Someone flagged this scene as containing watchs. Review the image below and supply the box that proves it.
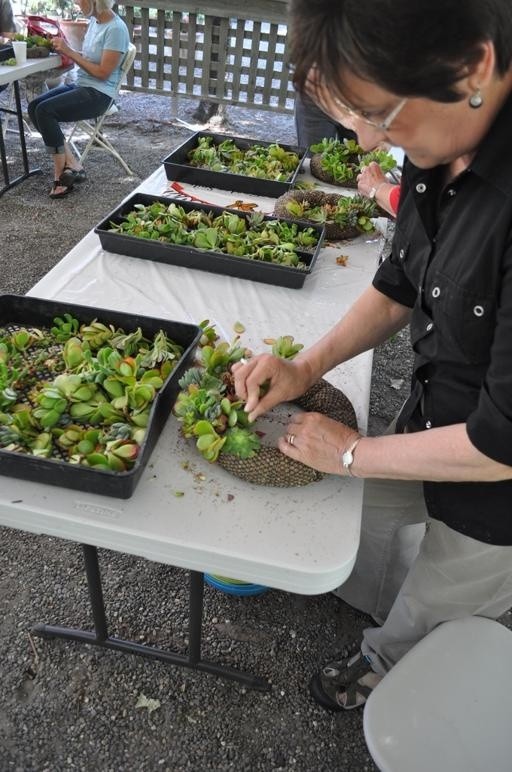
[340,439,361,478]
[369,182,382,201]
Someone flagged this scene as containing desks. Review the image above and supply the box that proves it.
[0,50,86,197]
[0,128,390,691]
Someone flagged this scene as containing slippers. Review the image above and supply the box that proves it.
[49,164,86,198]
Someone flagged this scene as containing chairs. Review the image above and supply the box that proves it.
[38,40,138,177]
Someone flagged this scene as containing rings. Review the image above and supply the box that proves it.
[287,434,295,445]
[240,358,248,365]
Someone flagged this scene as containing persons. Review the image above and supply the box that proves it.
[356,161,401,218]
[293,61,356,157]
[230,0,512,711]
[0,0,16,93]
[28,0,131,198]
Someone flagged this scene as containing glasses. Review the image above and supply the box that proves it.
[340,95,406,131]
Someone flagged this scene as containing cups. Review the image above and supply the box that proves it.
[12,41,28,65]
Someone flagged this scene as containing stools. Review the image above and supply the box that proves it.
[363,611,512,767]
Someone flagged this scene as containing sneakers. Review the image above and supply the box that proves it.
[310,651,381,711]
